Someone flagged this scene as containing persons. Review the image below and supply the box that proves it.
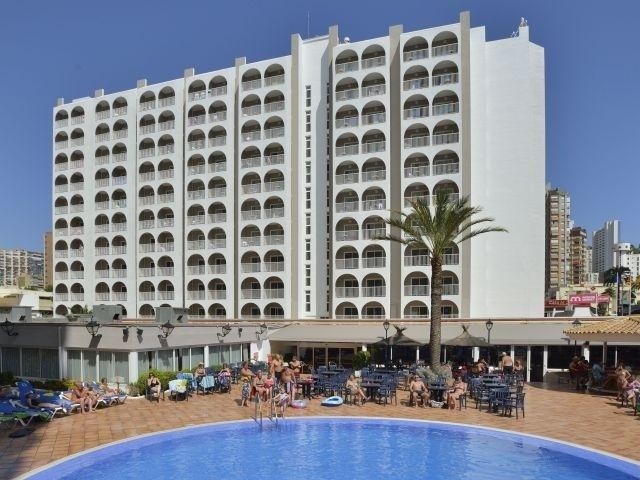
[448,376,467,410]
[409,374,428,409]
[25,393,60,408]
[147,372,161,404]
[346,374,370,405]
[456,352,526,384]
[562,355,640,411]
[275,388,290,419]
[71,376,126,414]
[195,354,302,406]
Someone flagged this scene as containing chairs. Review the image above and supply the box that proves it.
[236,360,527,419]
[622,386,640,416]
[0,364,236,433]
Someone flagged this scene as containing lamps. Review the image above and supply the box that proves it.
[85,315,100,338]
[0,317,19,336]
[256,322,267,335]
[217,322,232,337]
[158,320,175,339]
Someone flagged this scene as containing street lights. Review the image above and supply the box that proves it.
[383,317,390,362]
[486,317,493,364]
[572,318,582,389]
